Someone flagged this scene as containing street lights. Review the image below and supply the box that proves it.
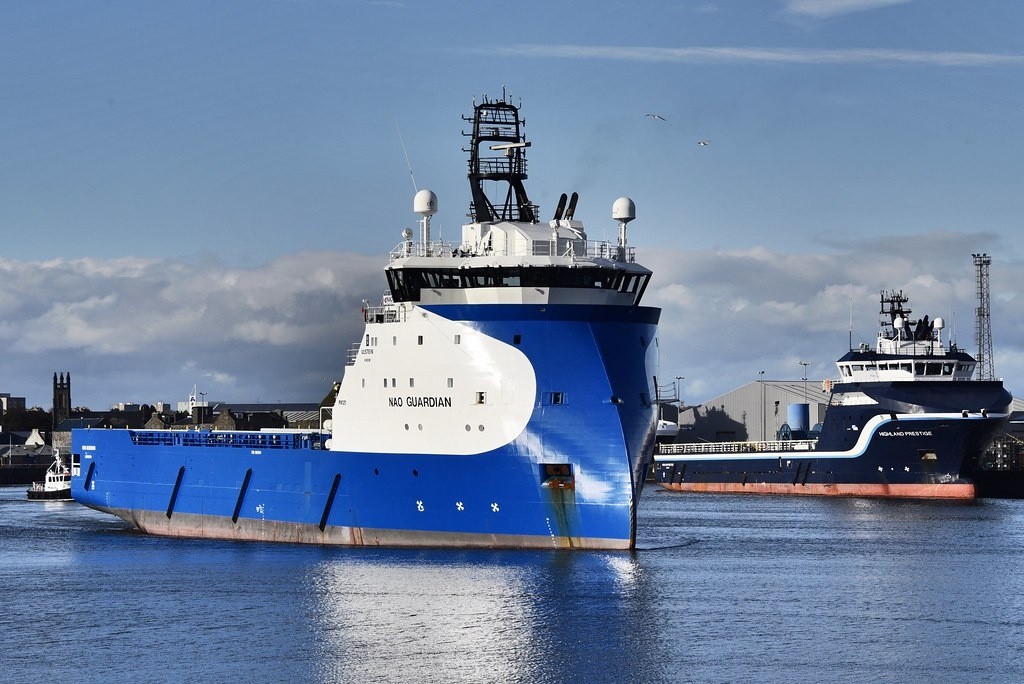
[757,370,766,442]
[199,392,208,430]
[798,360,812,402]
[673,375,684,443]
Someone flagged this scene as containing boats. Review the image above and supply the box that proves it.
[25,446,76,503]
[652,290,1013,504]
[68,81,669,557]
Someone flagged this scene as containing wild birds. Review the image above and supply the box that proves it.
[698,141,707,146]
[646,114,667,121]
